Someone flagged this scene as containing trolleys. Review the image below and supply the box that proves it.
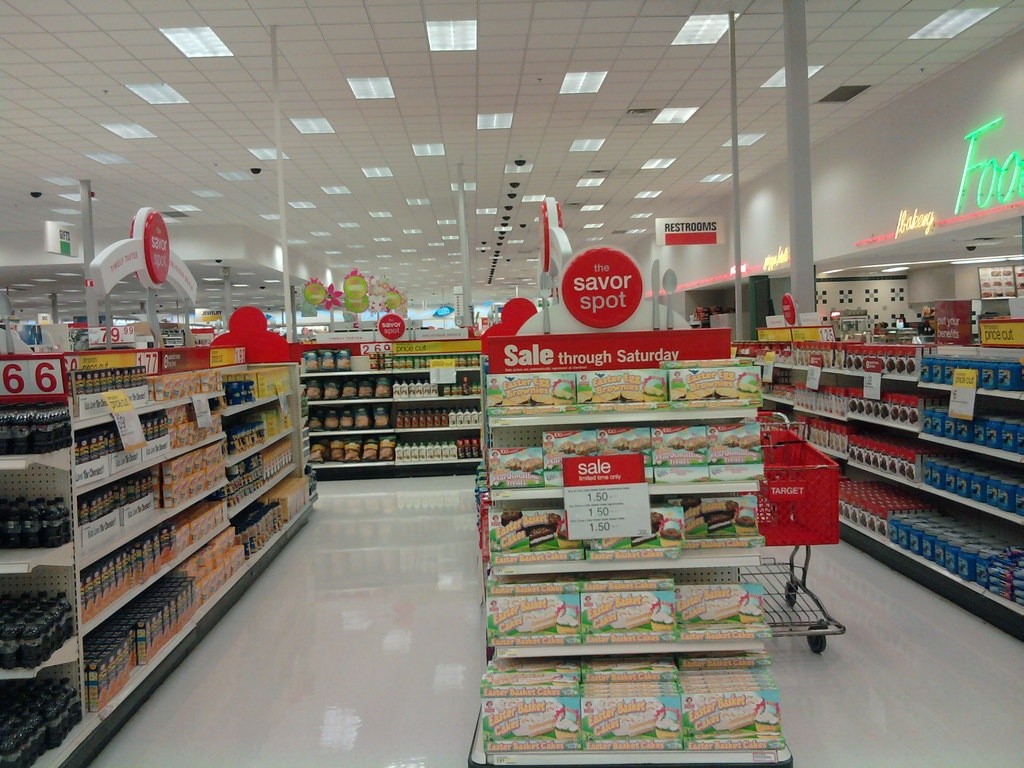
[737,412,846,654]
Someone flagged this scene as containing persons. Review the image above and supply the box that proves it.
[545,435,554,446]
[673,372,683,382]
[708,428,717,439]
[584,701,594,713]
[653,430,662,442]
[486,701,495,712]
[597,431,607,443]
[491,515,500,526]
[490,452,500,462]
[584,596,593,607]
[685,697,695,708]
[675,589,682,598]
[489,601,499,611]
[490,379,499,390]
[579,376,589,385]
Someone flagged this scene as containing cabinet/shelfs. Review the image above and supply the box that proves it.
[221,390,315,584]
[753,360,1024,641]
[300,370,394,481]
[247,363,318,505]
[393,368,483,478]
[0,447,86,768]
[72,389,251,733]
[466,405,793,768]
